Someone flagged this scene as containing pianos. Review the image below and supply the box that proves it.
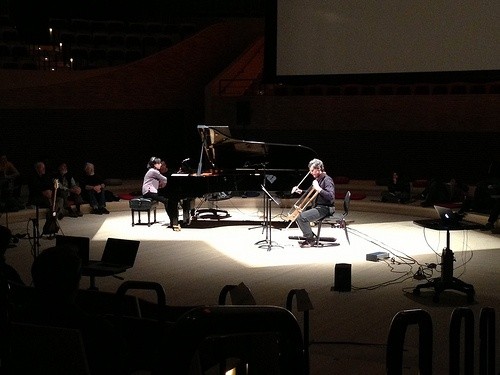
[166,125,316,233]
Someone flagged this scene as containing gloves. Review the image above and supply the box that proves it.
[312,180,322,193]
[291,186,302,194]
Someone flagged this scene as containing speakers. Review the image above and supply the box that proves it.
[335,263,352,292]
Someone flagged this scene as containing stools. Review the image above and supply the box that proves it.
[129,196,157,227]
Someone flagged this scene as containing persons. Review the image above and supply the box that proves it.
[16,245,128,375]
[28,162,64,219]
[80,163,109,214]
[142,157,184,228]
[291,159,336,248]
[49,162,83,217]
[383,171,402,203]
[0,226,26,318]
[0,152,25,211]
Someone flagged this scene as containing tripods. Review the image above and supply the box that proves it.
[247,174,284,249]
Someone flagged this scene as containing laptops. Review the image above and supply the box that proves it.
[434,205,471,225]
[90,238,141,269]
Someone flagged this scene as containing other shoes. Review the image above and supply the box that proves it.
[92,208,102,215]
[77,210,83,216]
[101,207,109,214]
[68,210,77,218]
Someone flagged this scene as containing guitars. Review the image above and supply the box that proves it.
[42,180,61,238]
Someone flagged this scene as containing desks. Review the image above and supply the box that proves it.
[415,219,486,301]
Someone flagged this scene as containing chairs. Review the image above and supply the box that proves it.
[55,236,140,292]
[317,191,351,247]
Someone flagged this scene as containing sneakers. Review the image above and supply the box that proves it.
[300,236,318,247]
